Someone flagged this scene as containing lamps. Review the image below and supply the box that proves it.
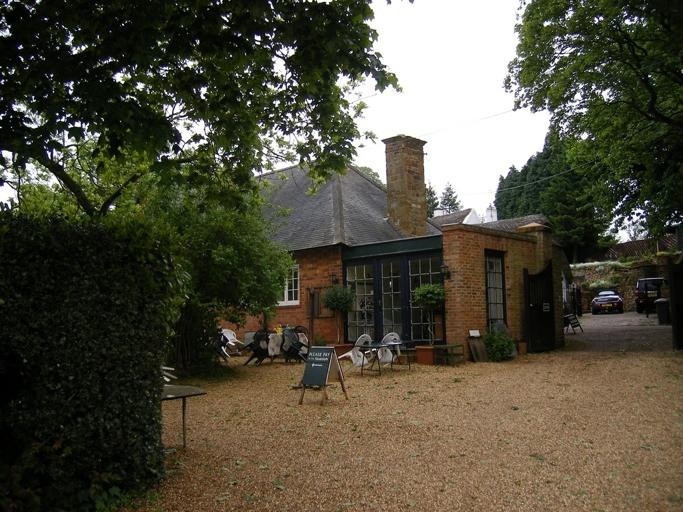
[439,264,451,279]
[329,272,339,283]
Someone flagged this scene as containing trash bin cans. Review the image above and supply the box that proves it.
[655,298,670,323]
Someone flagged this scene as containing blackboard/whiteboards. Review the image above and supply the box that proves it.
[302,346,334,386]
[567,314,579,327]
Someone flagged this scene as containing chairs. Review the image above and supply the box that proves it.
[215,325,311,367]
[337,332,403,376]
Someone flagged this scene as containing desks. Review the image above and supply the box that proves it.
[350,339,414,374]
